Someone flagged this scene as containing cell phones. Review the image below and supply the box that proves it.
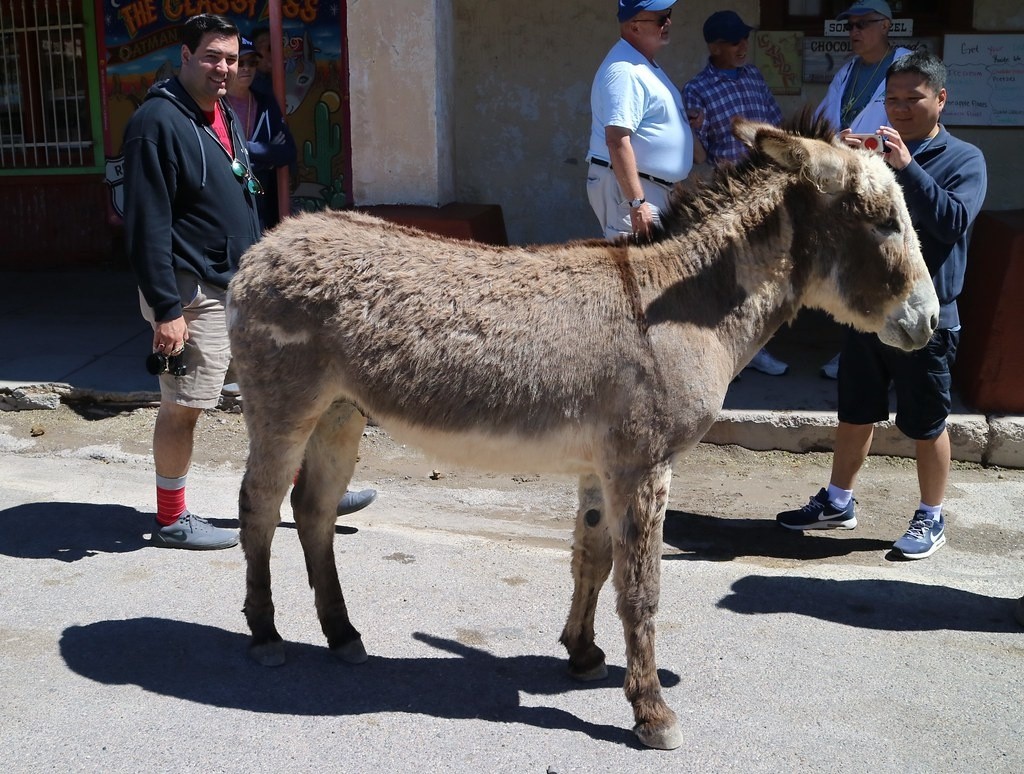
[847,133,884,153]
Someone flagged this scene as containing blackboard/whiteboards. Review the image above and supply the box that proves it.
[937,28,1024,130]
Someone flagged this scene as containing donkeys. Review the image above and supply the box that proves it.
[223,103,941,752]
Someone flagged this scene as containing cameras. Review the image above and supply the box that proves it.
[146,350,186,375]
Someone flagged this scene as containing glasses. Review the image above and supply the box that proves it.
[631,8,672,27]
[714,32,750,46]
[238,59,259,67]
[843,19,886,31]
[231,158,264,194]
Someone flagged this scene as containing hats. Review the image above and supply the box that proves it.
[239,37,262,57]
[703,11,754,41]
[617,0,678,23]
[837,0,892,21]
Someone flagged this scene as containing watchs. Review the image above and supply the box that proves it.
[629,197,645,208]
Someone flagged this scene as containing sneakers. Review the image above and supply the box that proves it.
[291,489,376,523]
[152,509,240,550]
[776,488,858,530]
[891,510,945,558]
[820,352,840,379]
[744,346,790,375]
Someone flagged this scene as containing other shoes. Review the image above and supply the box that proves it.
[221,375,241,395]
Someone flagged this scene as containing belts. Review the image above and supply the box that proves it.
[591,156,673,187]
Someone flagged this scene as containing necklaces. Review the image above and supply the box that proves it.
[844,41,889,121]
[226,90,251,141]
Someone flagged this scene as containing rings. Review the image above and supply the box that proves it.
[159,344,166,349]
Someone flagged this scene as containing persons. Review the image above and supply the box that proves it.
[681,10,788,374]
[586,0,704,240]
[222,32,294,395]
[775,52,987,559]
[122,13,376,550]
[811,0,915,380]
